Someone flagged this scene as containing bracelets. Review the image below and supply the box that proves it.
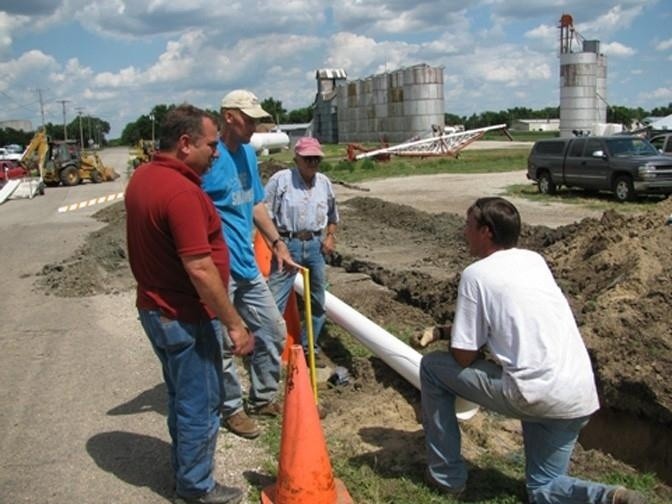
[272,238,283,246]
[435,325,444,340]
[326,232,337,239]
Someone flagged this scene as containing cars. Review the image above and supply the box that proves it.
[0,143,26,180]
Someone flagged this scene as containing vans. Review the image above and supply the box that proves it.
[527,136,672,201]
[643,134,672,157]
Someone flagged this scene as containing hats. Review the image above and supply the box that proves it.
[294,137,324,156]
[221,89,272,119]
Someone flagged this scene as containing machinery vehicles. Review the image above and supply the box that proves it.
[20,131,120,185]
[130,138,160,169]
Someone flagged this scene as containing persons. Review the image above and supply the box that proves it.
[410,194,644,504]
[197,88,289,437]
[263,136,339,369]
[124,104,243,504]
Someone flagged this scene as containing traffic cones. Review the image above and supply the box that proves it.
[254,227,301,363]
[261,348,355,504]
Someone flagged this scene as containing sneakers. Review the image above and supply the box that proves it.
[172,477,241,504]
[424,468,466,492]
[247,403,280,416]
[224,411,259,438]
[612,488,645,504]
[305,355,325,368]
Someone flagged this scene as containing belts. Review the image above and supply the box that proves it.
[280,232,320,240]
